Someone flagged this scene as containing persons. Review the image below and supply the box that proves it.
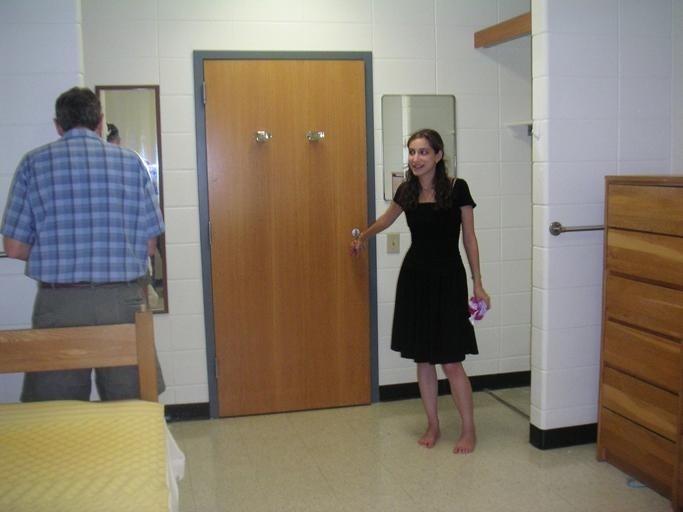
[352,130,490,455]
[106,123,158,202]
[0,87,166,402]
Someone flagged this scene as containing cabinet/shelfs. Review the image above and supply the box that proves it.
[593,174,682,506]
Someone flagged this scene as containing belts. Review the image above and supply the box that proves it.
[41,281,90,288]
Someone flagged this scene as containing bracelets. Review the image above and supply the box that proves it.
[471,273,480,280]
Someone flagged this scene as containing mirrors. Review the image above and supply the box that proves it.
[381,94,458,201]
[96,84,170,316]
